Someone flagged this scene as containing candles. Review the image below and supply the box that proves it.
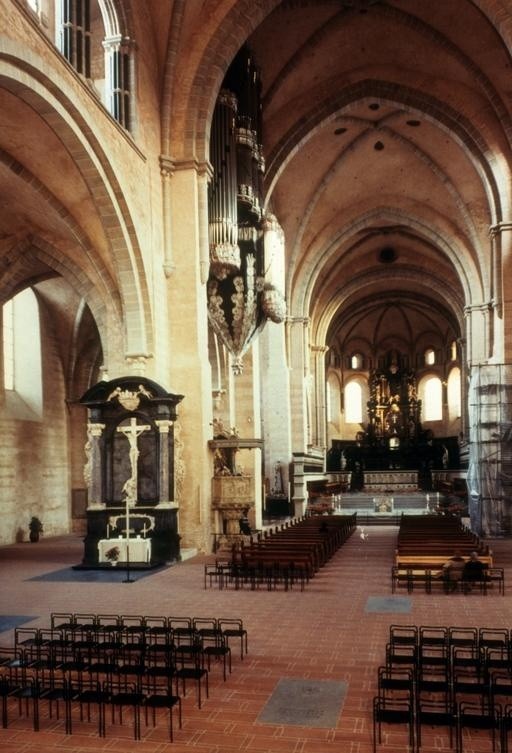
[106,524,110,540]
[125,494,130,549]
[143,522,147,537]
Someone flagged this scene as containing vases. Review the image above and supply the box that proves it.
[110,560,118,566]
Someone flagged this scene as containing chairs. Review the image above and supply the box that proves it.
[201,505,361,595]
[368,623,512,752]
[390,507,507,596]
[0,611,249,743]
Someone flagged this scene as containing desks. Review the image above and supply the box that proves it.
[96,535,154,566]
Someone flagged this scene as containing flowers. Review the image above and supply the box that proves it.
[104,546,120,561]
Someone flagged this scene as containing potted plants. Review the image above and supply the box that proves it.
[26,512,45,543]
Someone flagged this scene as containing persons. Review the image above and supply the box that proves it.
[440,548,466,592]
[460,551,486,592]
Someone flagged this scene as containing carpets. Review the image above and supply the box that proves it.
[21,563,176,583]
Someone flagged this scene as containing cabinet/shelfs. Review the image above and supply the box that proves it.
[69,374,184,573]
